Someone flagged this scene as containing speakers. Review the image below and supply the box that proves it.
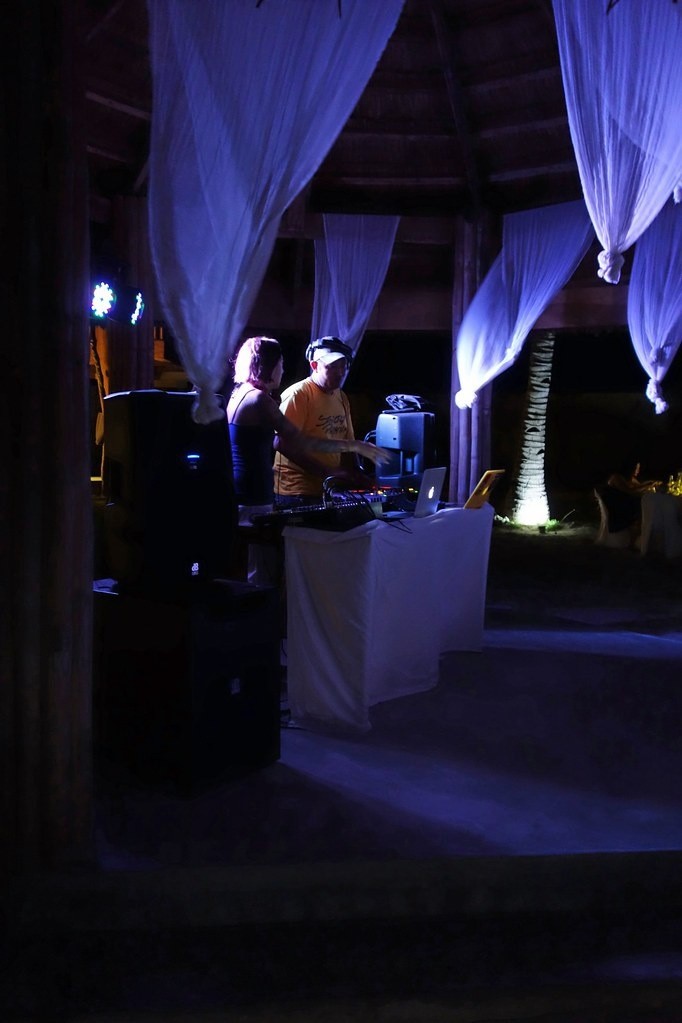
[375,412,437,492]
[103,390,236,603]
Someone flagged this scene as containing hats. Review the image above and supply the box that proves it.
[306,336,353,363]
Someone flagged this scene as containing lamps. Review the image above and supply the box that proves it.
[90,273,151,328]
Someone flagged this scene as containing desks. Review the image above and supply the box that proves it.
[282,504,491,730]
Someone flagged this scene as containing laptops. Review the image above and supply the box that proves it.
[462,469,506,509]
[383,467,446,520]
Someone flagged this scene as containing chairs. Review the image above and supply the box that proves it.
[593,483,637,546]
[639,494,682,559]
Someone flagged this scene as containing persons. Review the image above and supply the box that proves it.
[275,337,358,511]
[229,335,391,585]
[604,458,663,550]
